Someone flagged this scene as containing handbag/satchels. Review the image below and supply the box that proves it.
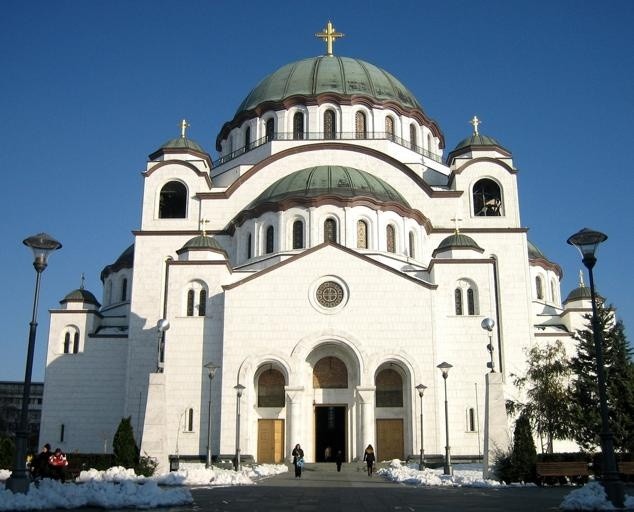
[296,457,304,467]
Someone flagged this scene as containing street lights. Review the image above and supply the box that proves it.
[415,384,427,471]
[5,232,62,495]
[437,362,453,476]
[234,384,246,472]
[204,362,221,470]
[567,227,625,508]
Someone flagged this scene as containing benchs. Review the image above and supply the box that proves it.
[607,462,634,483]
[27,457,81,483]
[536,461,590,489]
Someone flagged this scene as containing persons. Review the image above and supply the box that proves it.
[31,443,68,483]
[364,444,375,477]
[336,449,342,472]
[292,444,304,479]
[325,446,331,462]
